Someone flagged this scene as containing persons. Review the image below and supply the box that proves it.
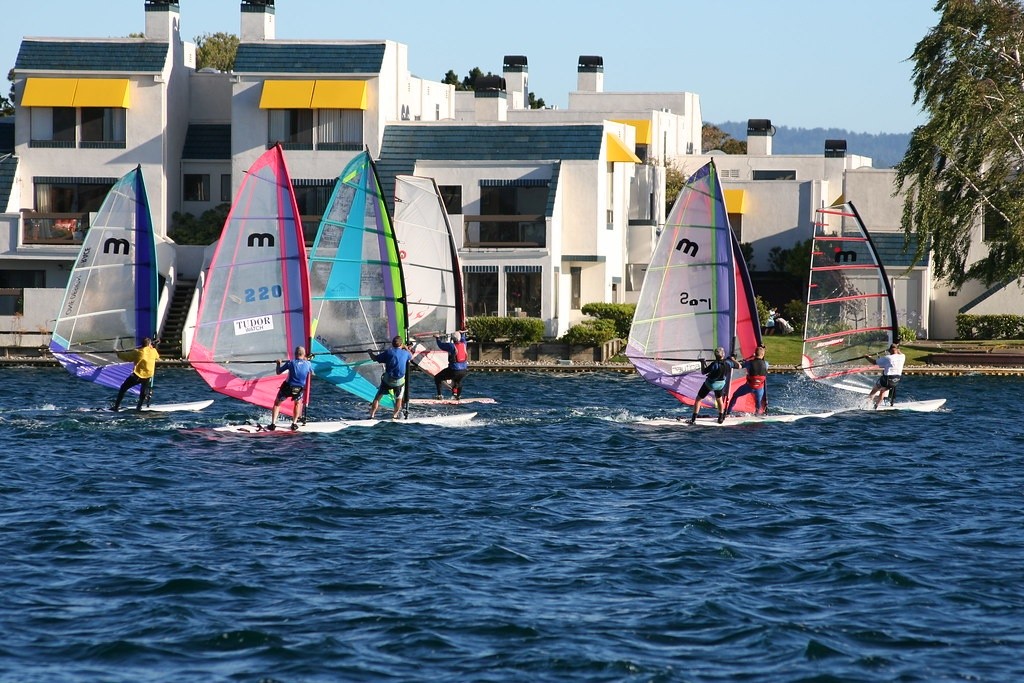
[367,336,412,420]
[432,330,469,400]
[267,346,311,430]
[764,307,780,336]
[727,346,770,415]
[685,346,742,424]
[109,337,160,412]
[863,344,906,409]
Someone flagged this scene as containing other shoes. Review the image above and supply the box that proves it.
[718,418,723,424]
[455,396,460,400]
[109,406,118,411]
[392,415,400,419]
[136,407,141,410]
[267,424,275,430]
[291,423,298,430]
[367,417,374,420]
[437,395,442,400]
[685,420,693,424]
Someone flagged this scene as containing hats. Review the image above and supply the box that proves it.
[451,331,461,341]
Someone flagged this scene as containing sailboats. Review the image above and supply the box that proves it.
[776,201,948,417]
[296,141,480,430]
[621,157,837,428]
[46,163,215,417]
[382,173,497,405]
[178,142,314,442]
